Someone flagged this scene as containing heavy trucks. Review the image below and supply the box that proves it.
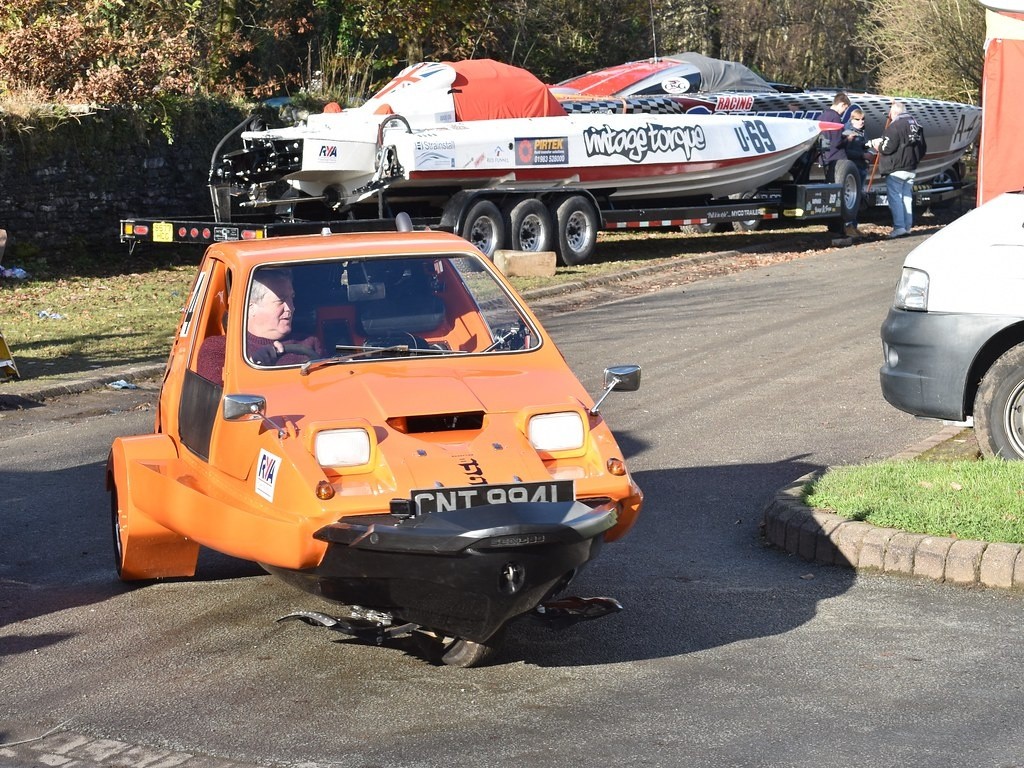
[117,49,981,269]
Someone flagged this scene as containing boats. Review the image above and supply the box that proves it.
[302,52,985,209]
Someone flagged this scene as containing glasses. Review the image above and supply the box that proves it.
[854,117,866,121]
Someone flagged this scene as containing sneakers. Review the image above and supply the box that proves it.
[844,225,860,237]
[856,230,868,238]
[906,230,911,235]
[886,228,906,238]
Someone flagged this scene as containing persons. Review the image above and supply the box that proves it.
[815,94,928,247]
[196,271,343,385]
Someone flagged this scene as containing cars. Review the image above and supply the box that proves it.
[876,185,1024,468]
